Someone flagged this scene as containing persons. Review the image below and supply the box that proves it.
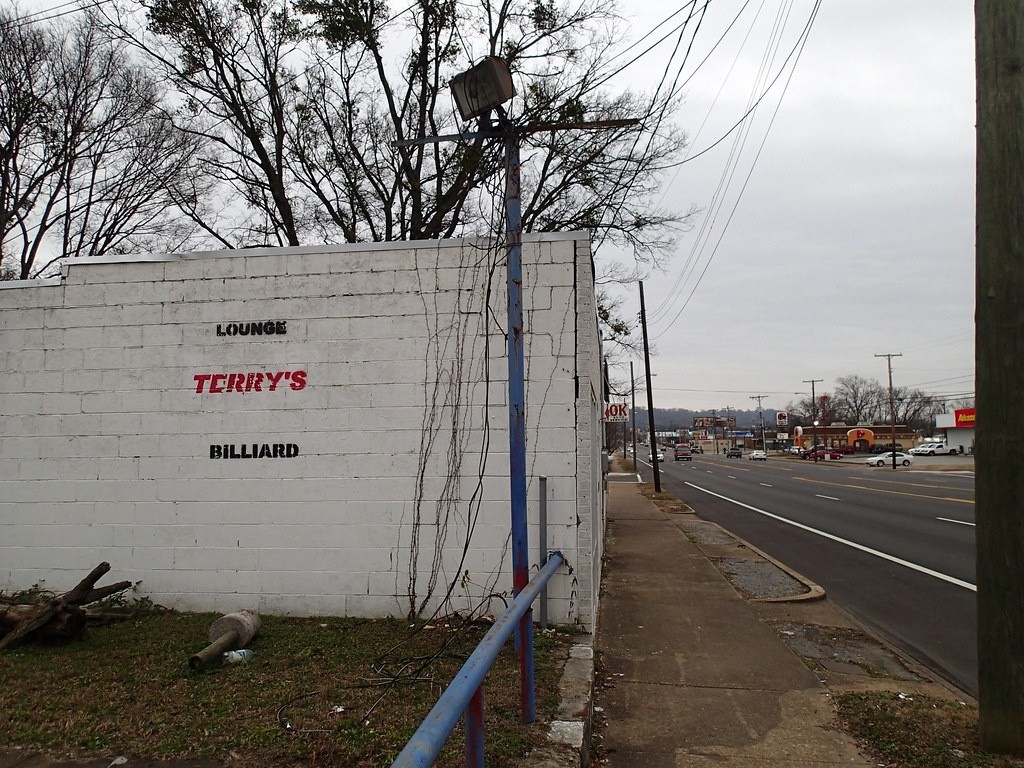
[723,448,726,454]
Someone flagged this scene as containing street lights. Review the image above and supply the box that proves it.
[630,361,658,472]
[795,392,819,462]
[448,53,532,651]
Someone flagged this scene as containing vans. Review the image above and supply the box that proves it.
[674,444,693,461]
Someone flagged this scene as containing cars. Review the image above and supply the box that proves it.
[865,451,916,467]
[648,448,665,462]
[784,440,961,461]
[748,449,767,461]
[626,442,666,454]
[726,446,742,458]
